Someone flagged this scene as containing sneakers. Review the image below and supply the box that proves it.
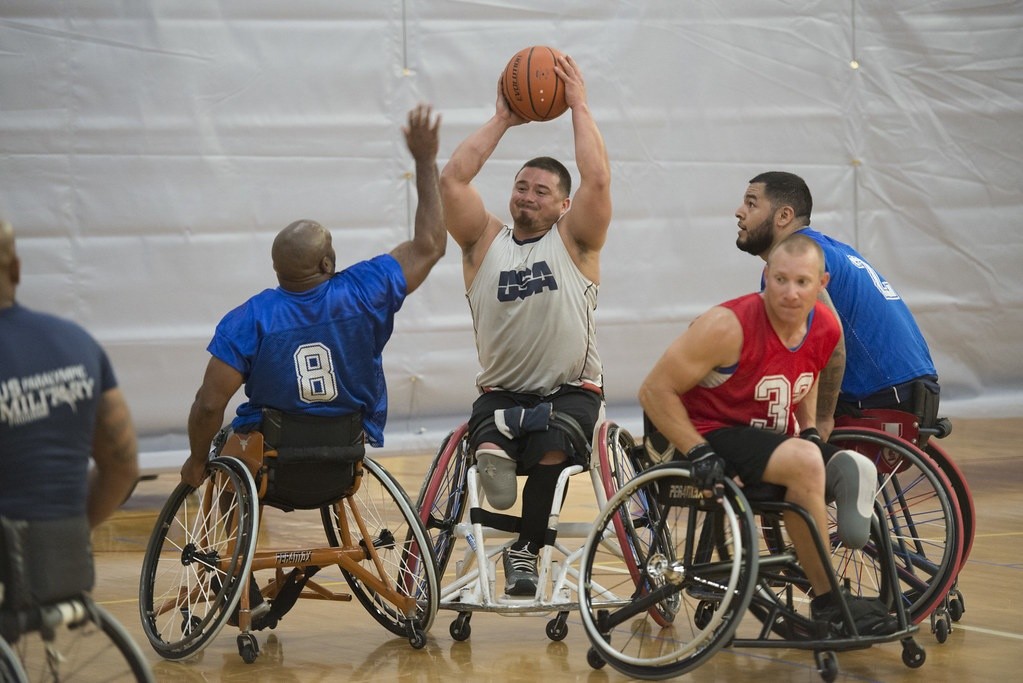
[811,586,900,638]
[503,546,540,596]
[227,571,270,626]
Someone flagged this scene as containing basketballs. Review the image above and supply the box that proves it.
[499,44,572,124]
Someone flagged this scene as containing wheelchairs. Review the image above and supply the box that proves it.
[397,376,684,641]
[0,474,158,683]
[576,378,977,683]
[139,406,441,663]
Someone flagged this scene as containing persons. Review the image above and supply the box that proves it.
[736,172,939,446]
[0,217,138,601]
[437,55,611,595]
[180,104,448,622]
[641,234,898,637]
